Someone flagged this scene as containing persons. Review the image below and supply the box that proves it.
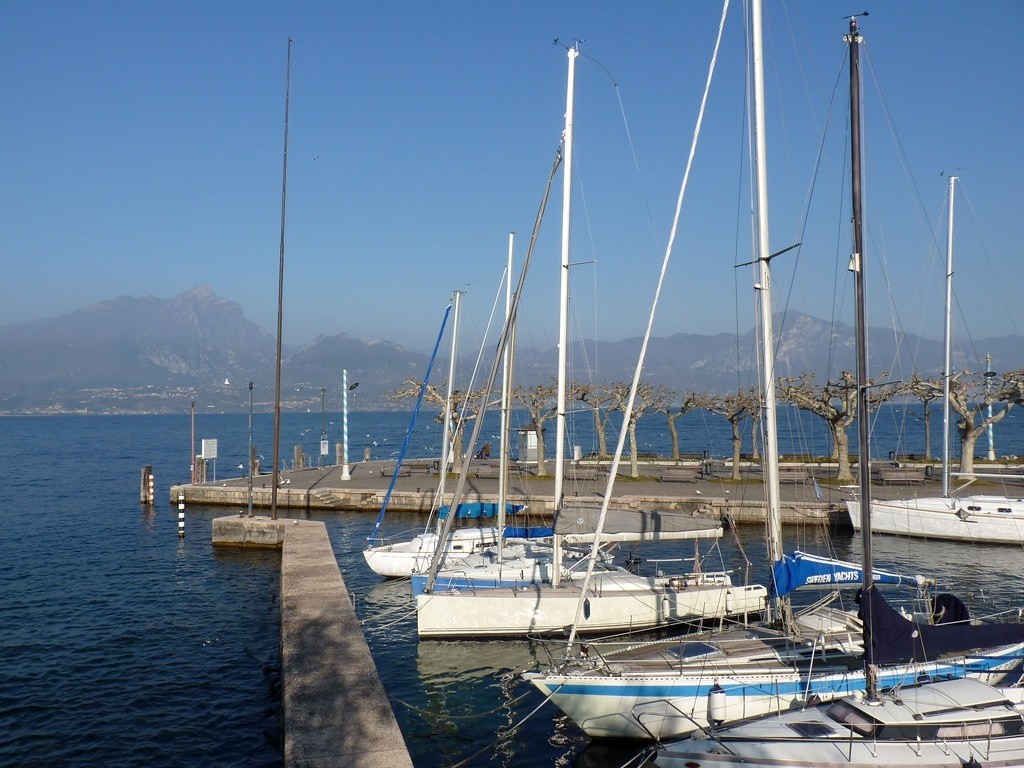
[477,440,489,459]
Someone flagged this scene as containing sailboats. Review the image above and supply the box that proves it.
[364,0,1024,767]
[844,174,1024,548]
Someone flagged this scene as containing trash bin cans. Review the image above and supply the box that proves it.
[704,451,708,460]
[888,450,894,460]
[574,446,582,461]
[926,465,933,477]
[706,463,712,475]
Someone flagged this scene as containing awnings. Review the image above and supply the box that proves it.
[438,502,528,520]
